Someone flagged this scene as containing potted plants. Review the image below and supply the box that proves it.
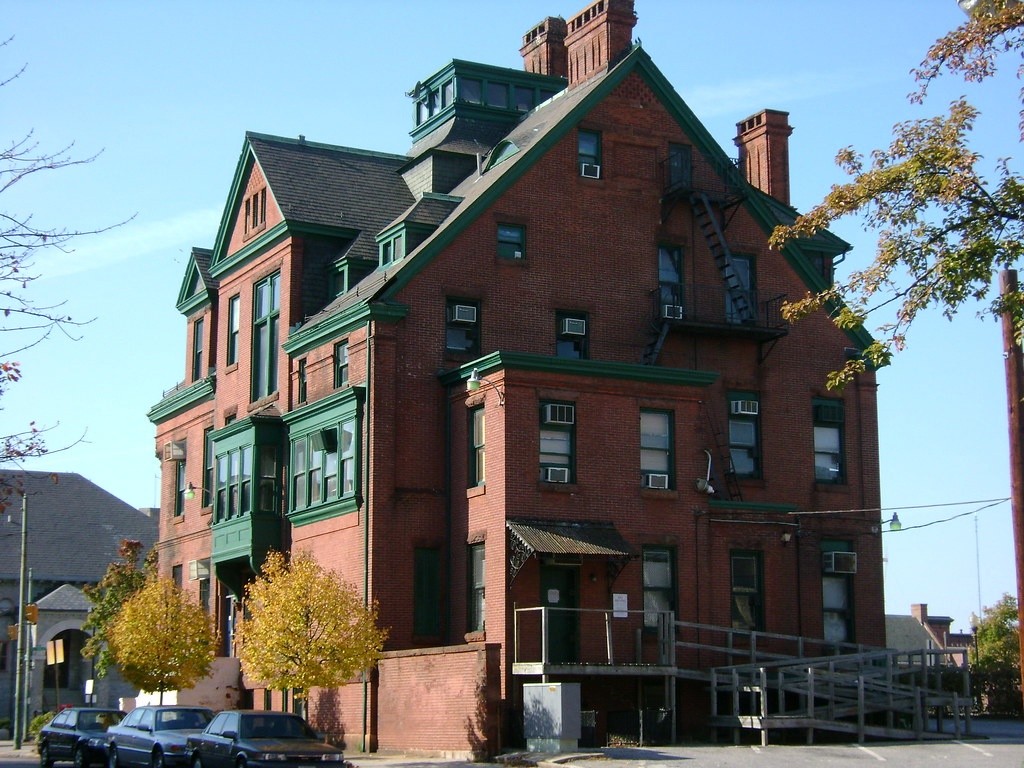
[609,730,649,747]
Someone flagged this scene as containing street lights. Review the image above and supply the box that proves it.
[969,611,984,715]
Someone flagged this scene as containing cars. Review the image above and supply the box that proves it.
[106,705,217,768]
[187,710,344,768]
[37,708,129,768]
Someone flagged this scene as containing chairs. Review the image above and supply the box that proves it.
[269,719,290,736]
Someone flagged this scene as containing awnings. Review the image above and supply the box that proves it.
[509,523,640,588]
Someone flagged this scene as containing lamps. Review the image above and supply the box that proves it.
[871,511,903,534]
[590,573,597,582]
[695,448,715,494]
[183,480,211,500]
[465,363,506,406]
[779,531,792,548]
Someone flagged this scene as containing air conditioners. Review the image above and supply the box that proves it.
[164,440,186,462]
[547,467,570,482]
[648,474,668,489]
[563,317,586,335]
[826,551,858,575]
[185,558,210,581]
[731,400,760,416]
[664,305,683,319]
[452,305,478,324]
[581,163,600,179]
[546,405,574,425]
